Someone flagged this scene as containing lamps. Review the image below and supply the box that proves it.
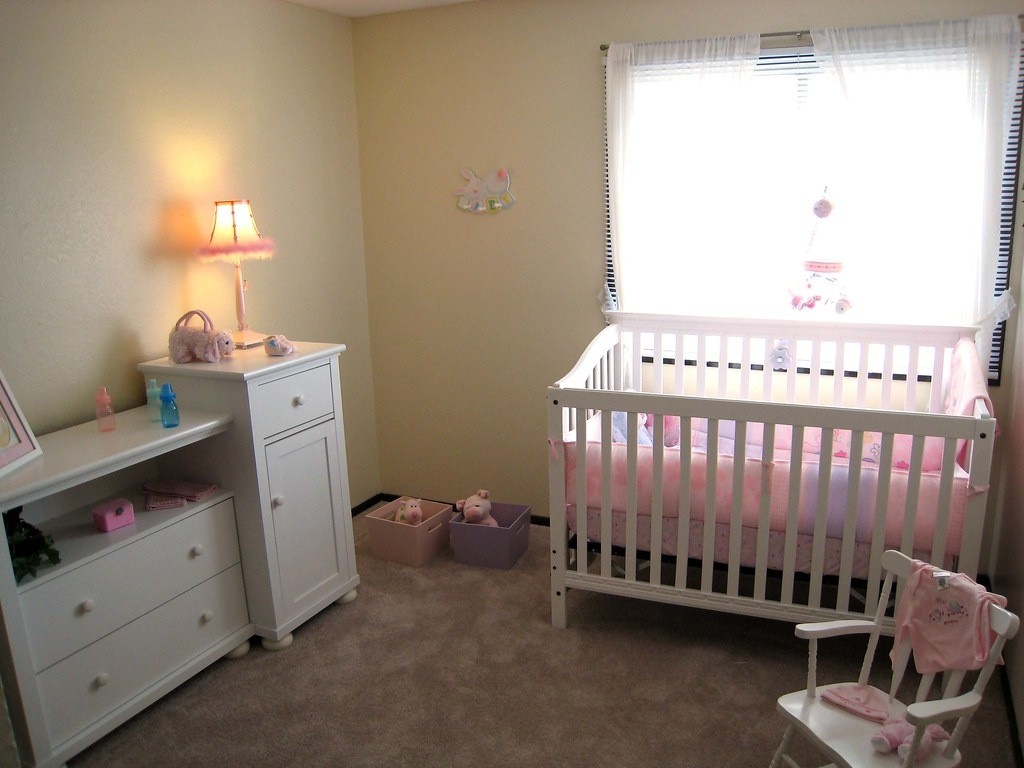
[194,200,274,348]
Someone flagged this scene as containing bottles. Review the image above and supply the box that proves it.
[95,387,116,432]
[160,383,179,428]
[145,379,162,421]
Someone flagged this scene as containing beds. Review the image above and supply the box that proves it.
[544,304,998,637]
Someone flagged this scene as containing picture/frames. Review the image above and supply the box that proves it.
[0,371,47,479]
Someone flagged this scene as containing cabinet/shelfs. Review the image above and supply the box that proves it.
[0,337,364,768]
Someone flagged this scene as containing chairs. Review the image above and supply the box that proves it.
[770,549,1022,768]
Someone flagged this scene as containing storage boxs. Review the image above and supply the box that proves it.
[93,497,135,534]
[363,494,454,569]
[447,493,536,571]
